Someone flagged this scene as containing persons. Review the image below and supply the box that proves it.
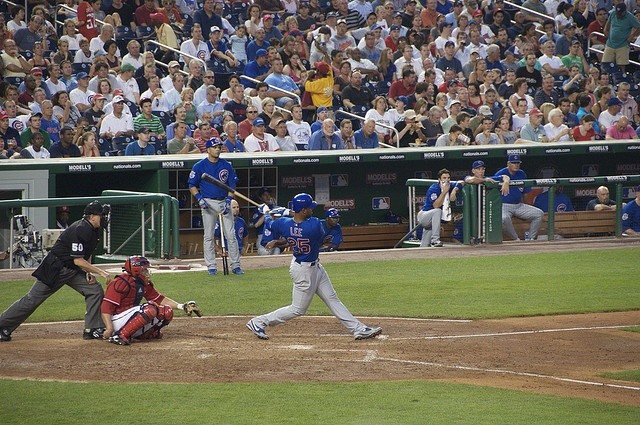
[495,154,544,241]
[187,139,245,276]
[101,256,203,345]
[1,0,640,158]
[622,186,640,236]
[247,193,382,339]
[417,168,466,248]
[258,207,287,255]
[214,199,248,255]
[250,187,287,255]
[320,207,344,251]
[586,186,628,211]
[465,160,510,196]
[1,202,111,341]
[51,206,70,229]
[533,184,574,212]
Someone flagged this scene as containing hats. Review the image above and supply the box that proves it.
[395,95,408,106]
[77,71,91,82]
[615,4,627,16]
[326,11,338,19]
[254,118,264,127]
[372,24,383,31]
[470,49,478,56]
[0,111,9,122]
[608,97,623,106]
[392,12,402,18]
[29,112,43,120]
[166,61,180,68]
[121,64,137,73]
[151,12,166,25]
[263,14,272,21]
[448,80,459,86]
[113,89,124,95]
[472,10,483,17]
[209,26,220,33]
[528,108,542,117]
[403,109,415,120]
[93,93,107,101]
[114,96,125,104]
[407,1,417,6]
[564,23,575,29]
[137,128,151,134]
[256,49,269,57]
[479,105,493,117]
[32,67,44,77]
[454,1,463,7]
[313,61,330,74]
[570,40,581,46]
[335,19,346,26]
[316,106,327,114]
[289,29,303,36]
[57,207,70,214]
[449,100,460,108]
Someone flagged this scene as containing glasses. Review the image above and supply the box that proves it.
[204,76,215,79]
[247,111,258,113]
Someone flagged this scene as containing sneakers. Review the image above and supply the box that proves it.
[232,267,243,275]
[83,328,106,341]
[107,332,123,345]
[354,327,382,341]
[431,240,442,247]
[146,330,162,338]
[246,319,269,340]
[0,328,14,341]
[207,269,217,275]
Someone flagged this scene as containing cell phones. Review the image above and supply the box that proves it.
[314,70,318,75]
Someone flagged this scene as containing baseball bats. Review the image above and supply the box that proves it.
[218,214,229,275]
[201,173,261,208]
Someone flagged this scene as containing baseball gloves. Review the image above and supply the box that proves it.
[183,300,202,317]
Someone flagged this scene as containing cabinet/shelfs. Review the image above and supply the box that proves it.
[170,165,280,230]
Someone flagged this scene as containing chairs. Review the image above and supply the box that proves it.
[232,2,249,20]
[634,69,640,84]
[72,62,93,77]
[164,122,193,143]
[611,71,633,86]
[206,60,227,73]
[265,12,280,25]
[299,59,310,69]
[5,76,26,87]
[376,81,394,97]
[628,84,639,97]
[114,25,137,39]
[14,51,33,60]
[43,50,56,62]
[282,12,296,21]
[154,148,167,154]
[597,61,619,75]
[146,136,161,149]
[319,1,332,10]
[229,17,240,27]
[179,14,193,26]
[69,49,78,60]
[554,74,570,80]
[218,2,232,19]
[362,80,375,96]
[553,81,564,98]
[351,105,369,117]
[96,137,111,150]
[101,150,114,156]
[113,135,134,149]
[136,25,156,39]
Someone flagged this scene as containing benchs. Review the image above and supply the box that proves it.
[335,210,622,249]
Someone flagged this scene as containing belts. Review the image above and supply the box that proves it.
[295,260,319,266]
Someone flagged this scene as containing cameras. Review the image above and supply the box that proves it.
[566,128,571,133]
[580,73,589,80]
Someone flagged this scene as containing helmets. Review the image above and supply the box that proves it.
[124,256,149,285]
[508,156,523,163]
[326,208,341,219]
[472,160,486,169]
[258,187,271,196]
[83,201,112,232]
[293,193,317,213]
[192,217,203,228]
[207,138,221,148]
